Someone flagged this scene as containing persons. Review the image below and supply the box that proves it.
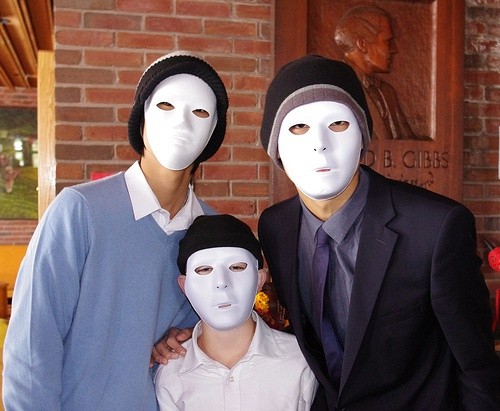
[334,5,418,142]
[153,214,322,410]
[2,50,229,411]
[147,55,500,410]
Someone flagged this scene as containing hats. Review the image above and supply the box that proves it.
[177,215,264,275]
[127,50,229,162]
[259,53,373,171]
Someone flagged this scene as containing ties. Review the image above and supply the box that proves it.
[311,224,342,400]
